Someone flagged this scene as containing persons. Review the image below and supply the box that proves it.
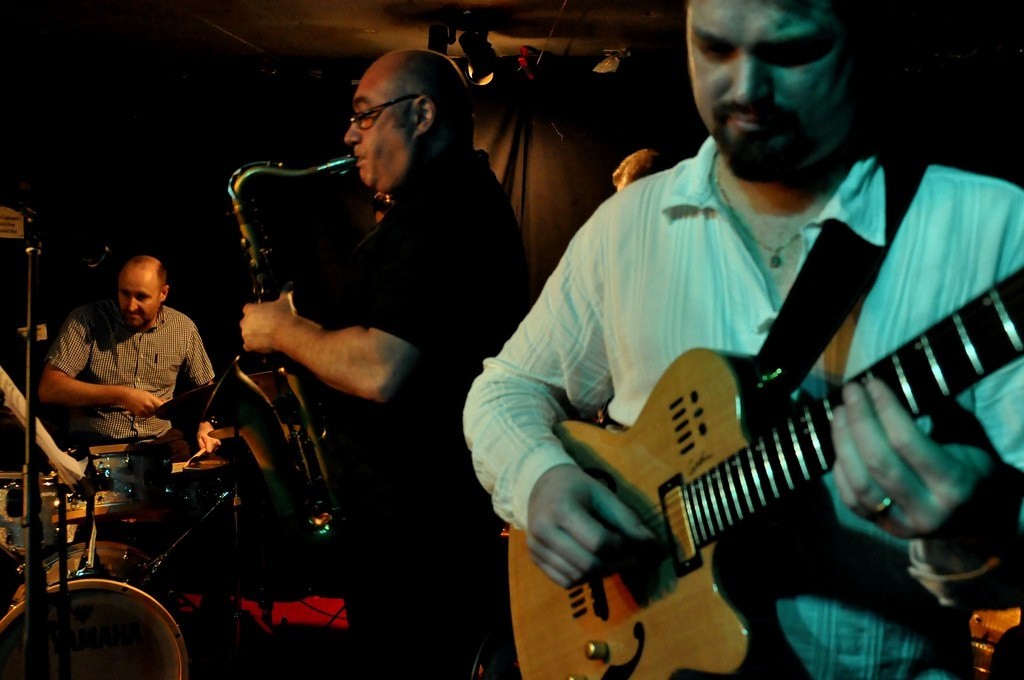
[39,254,235,652]
[462,0,1024,680]
[239,50,530,680]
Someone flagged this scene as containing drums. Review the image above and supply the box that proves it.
[0,440,190,680]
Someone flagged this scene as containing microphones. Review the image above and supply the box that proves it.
[17,205,112,270]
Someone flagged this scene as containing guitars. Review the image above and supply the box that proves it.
[509,266,1024,680]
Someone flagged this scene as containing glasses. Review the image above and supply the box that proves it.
[345,94,420,129]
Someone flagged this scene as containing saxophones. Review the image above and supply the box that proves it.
[204,152,360,546]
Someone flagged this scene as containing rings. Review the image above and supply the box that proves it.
[869,494,894,516]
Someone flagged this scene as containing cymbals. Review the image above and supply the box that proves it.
[206,424,327,446]
[155,370,288,422]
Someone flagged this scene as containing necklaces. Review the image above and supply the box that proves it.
[715,174,801,267]
